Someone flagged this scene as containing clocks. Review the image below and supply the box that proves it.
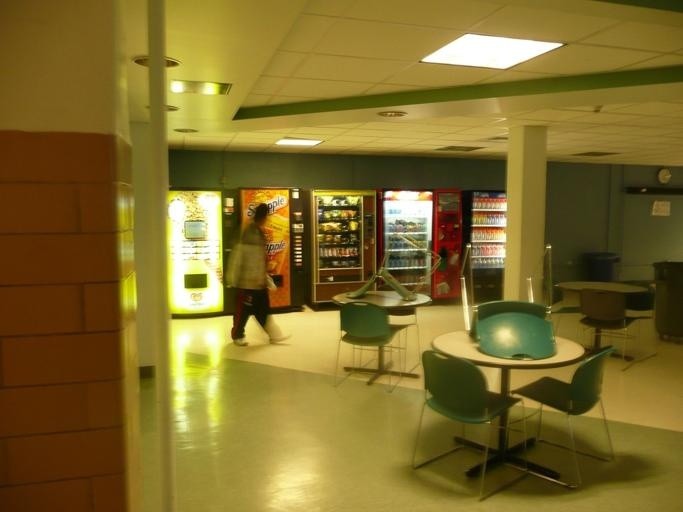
[656,168,673,184]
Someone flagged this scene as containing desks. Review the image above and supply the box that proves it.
[332,291,432,386]
[431,329,586,480]
[553,280,649,362]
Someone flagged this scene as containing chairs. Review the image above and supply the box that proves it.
[335,302,409,393]
[511,344,617,488]
[579,289,636,372]
[459,244,560,360]
[344,232,441,302]
[411,351,528,501]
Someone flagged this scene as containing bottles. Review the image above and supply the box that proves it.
[472,199,508,264]
[385,202,427,284]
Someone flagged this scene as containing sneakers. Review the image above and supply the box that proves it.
[269,332,292,344]
[232,337,249,346]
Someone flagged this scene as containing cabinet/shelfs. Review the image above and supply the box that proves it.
[385,211,428,287]
[318,205,360,262]
[470,207,506,259]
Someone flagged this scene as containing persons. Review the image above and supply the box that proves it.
[224,204,290,347]
